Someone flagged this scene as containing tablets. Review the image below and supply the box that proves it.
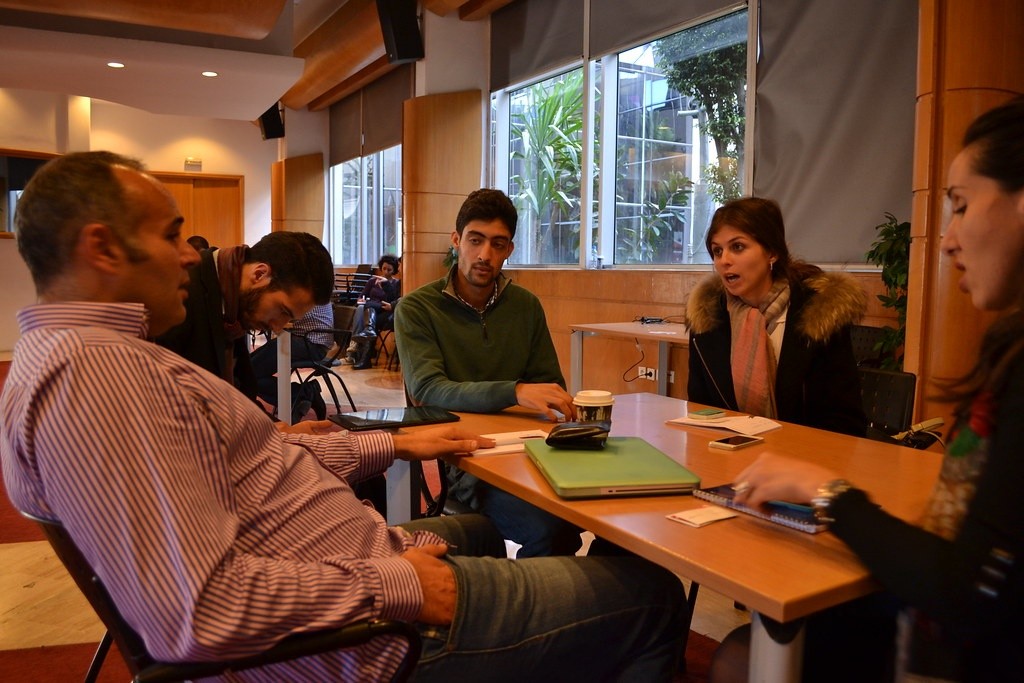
[327,405,460,432]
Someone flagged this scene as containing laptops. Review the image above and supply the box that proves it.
[522,435,701,500]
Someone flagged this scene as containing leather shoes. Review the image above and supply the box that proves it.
[291,397,312,426]
[309,379,326,421]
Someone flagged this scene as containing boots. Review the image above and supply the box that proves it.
[351,342,378,369]
[351,307,377,342]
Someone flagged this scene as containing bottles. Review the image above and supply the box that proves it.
[590,245,597,268]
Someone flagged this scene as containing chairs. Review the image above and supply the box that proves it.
[37,262,921,683]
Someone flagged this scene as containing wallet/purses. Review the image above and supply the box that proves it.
[547,418,611,451]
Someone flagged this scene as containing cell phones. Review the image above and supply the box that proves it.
[708,433,765,451]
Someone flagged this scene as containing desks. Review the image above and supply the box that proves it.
[567,323,689,396]
[381,391,944,683]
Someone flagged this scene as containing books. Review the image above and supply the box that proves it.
[695,482,829,533]
[687,408,725,418]
[466,429,549,457]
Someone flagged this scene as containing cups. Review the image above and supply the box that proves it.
[572,390,616,437]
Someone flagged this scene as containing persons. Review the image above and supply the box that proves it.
[684,197,865,437]
[352,257,401,368]
[338,255,398,364]
[235,301,333,424]
[186,236,209,252]
[147,232,333,397]
[0,151,693,682]
[711,99,1024,683]
[395,187,626,560]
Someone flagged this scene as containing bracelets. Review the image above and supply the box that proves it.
[812,478,851,521]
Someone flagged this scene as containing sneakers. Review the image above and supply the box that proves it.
[339,341,358,365]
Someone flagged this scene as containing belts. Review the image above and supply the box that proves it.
[390,633,423,683]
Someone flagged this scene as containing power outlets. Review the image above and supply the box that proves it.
[638,367,675,383]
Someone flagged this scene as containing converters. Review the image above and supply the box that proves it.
[643,317,663,323]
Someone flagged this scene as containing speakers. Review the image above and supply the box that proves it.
[258,102,285,140]
[375,1,425,63]
